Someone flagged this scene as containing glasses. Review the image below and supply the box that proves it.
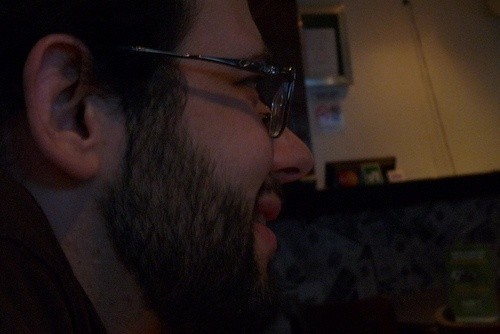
[92,44,293,137]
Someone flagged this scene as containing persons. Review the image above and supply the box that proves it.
[0,0,316,334]
